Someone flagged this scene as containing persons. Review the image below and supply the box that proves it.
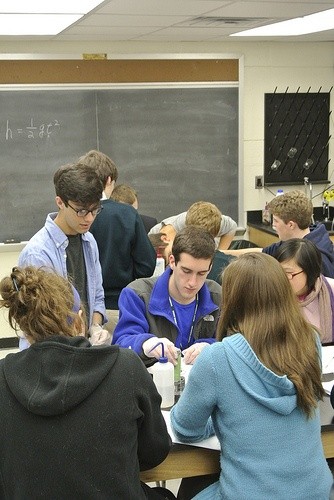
[169,251,334,500]
[0,264,178,500]
[17,150,334,365]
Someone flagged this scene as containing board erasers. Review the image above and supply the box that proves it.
[5,239,21,244]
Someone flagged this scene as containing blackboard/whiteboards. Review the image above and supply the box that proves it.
[0,83,245,251]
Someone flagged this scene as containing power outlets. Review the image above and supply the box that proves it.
[255,176,263,189]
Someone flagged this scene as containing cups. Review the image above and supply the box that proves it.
[173,376,185,395]
[261,209,271,226]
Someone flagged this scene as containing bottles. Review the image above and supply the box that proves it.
[276,189,284,197]
[153,254,165,276]
[322,197,329,219]
[149,342,175,408]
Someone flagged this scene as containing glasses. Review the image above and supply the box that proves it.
[64,200,104,217]
[285,270,304,280]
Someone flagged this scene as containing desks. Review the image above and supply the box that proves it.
[136,343,334,487]
[248,220,334,249]
